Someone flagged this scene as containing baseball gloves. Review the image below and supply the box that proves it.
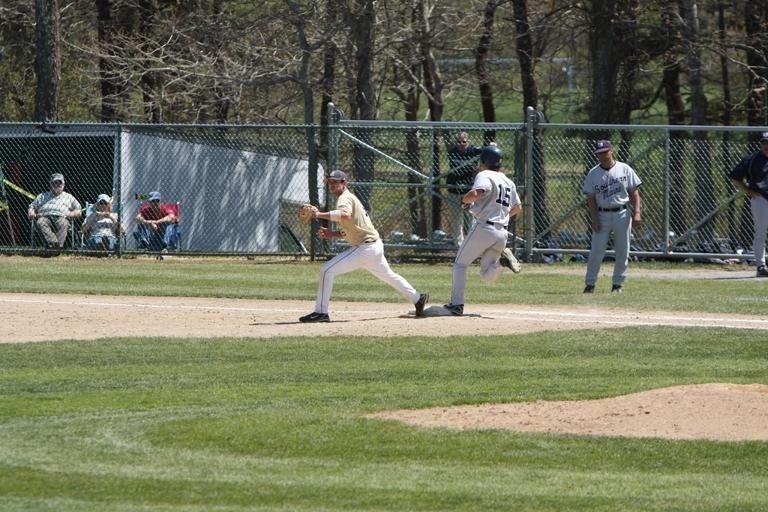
[298,204,319,225]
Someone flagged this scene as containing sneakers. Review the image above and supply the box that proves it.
[612,285,621,293]
[756,265,768,277]
[584,285,594,293]
[415,294,429,316]
[444,303,464,316]
[500,248,521,273]
[300,312,330,323]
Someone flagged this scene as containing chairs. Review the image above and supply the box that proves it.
[18,194,184,257]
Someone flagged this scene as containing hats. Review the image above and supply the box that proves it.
[595,141,611,154]
[51,174,63,182]
[326,170,347,181]
[147,192,160,201]
[97,194,109,203]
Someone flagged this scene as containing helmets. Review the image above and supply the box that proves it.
[481,146,503,169]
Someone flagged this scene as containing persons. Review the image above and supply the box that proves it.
[582,139,645,293]
[296,169,429,325]
[445,132,502,253]
[443,145,522,315]
[27,174,83,253]
[727,132,768,279]
[134,190,183,257]
[83,192,127,255]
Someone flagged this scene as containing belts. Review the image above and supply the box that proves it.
[598,205,626,211]
[486,220,494,225]
